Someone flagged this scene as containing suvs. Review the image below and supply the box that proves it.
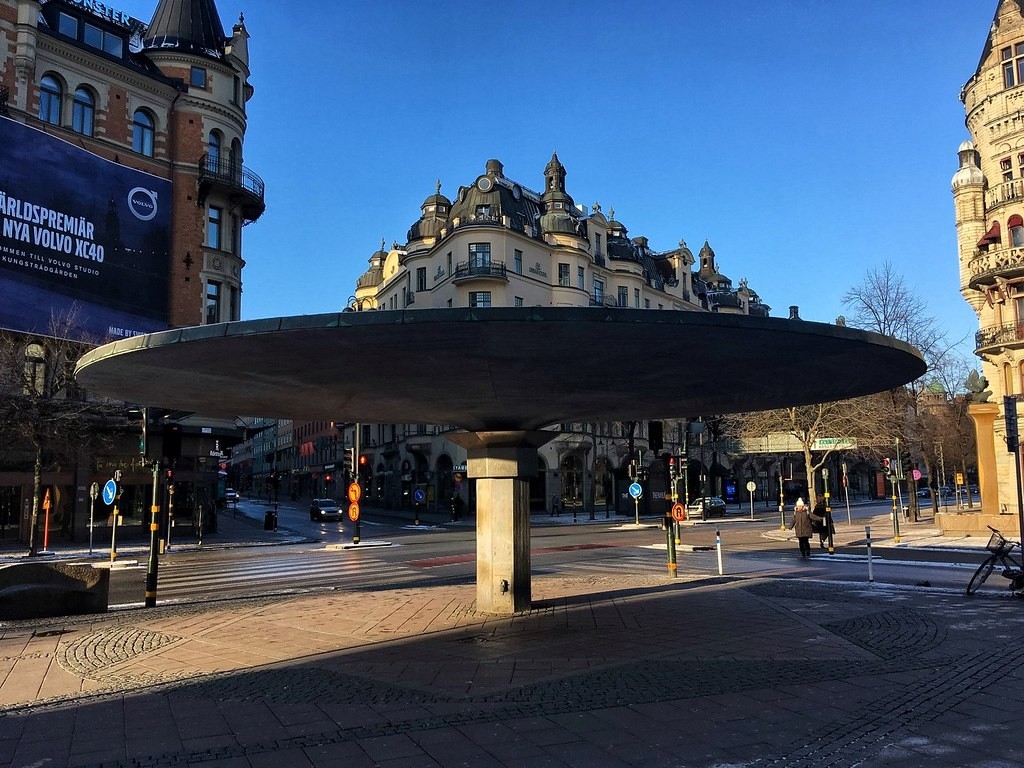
[684,497,726,519]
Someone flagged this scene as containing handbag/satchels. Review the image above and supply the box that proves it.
[811,520,818,533]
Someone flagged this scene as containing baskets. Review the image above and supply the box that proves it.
[986,533,1014,558]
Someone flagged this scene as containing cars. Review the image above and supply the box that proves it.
[969,485,979,494]
[936,486,952,497]
[226,487,240,502]
[957,486,967,495]
[917,487,936,498]
[309,498,343,522]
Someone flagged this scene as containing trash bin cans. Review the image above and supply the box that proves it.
[264,511,275,531]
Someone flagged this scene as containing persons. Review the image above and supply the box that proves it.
[812,496,836,548]
[455,492,464,518]
[789,497,823,558]
[61,505,71,538]
[551,492,559,517]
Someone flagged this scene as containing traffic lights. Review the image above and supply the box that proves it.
[882,457,891,475]
[900,450,912,473]
[358,455,368,465]
[344,447,355,473]
[842,476,849,488]
[821,469,829,478]
[678,447,687,475]
[165,469,174,490]
[326,476,331,481]
[670,458,678,481]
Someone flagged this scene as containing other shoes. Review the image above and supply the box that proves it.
[806,550,810,556]
[801,553,805,558]
[820,544,825,549]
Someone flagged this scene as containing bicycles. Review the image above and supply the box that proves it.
[967,526,1024,595]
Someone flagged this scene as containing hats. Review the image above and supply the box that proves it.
[796,497,804,506]
